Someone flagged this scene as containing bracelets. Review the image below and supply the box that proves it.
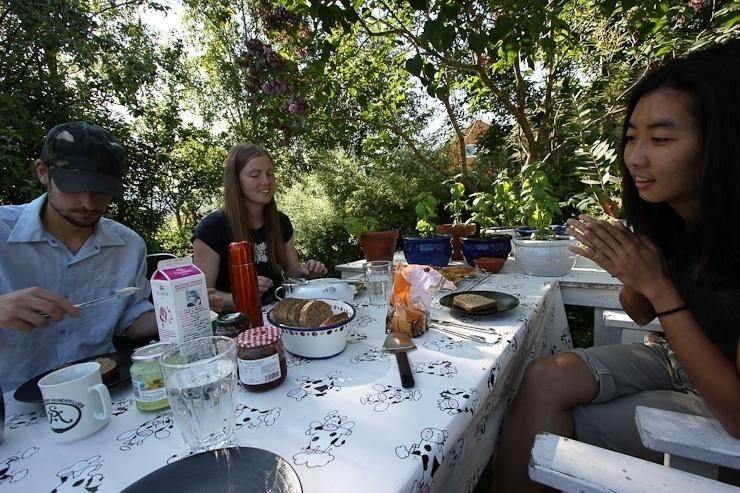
[650,305,687,321]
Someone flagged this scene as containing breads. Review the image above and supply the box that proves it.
[273,297,349,327]
[94,357,118,381]
[453,294,496,311]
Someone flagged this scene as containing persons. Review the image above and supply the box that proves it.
[191,142,328,312]
[1,122,223,394]
[492,39,740,492]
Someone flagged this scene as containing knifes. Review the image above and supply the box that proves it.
[40,289,135,318]
[425,318,496,342]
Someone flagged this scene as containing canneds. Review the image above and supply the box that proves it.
[235,326,287,392]
[214,311,249,338]
[129,341,186,413]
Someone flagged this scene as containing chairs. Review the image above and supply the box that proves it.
[600,304,740,481]
[144,252,180,281]
[527,431,740,493]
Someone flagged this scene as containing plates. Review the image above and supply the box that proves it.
[439,290,521,316]
[14,350,152,405]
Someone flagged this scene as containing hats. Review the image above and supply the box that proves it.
[40,120,120,199]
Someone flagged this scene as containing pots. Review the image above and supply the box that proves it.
[289,278,358,310]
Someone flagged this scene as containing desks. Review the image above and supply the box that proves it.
[336,244,654,345]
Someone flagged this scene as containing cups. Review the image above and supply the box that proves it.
[160,333,241,452]
[361,260,398,307]
[274,277,308,300]
[36,360,112,447]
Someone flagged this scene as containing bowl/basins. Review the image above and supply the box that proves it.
[474,257,505,274]
[268,296,354,363]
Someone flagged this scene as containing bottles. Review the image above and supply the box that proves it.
[227,240,264,329]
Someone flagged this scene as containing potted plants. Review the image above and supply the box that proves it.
[346,160,581,279]
